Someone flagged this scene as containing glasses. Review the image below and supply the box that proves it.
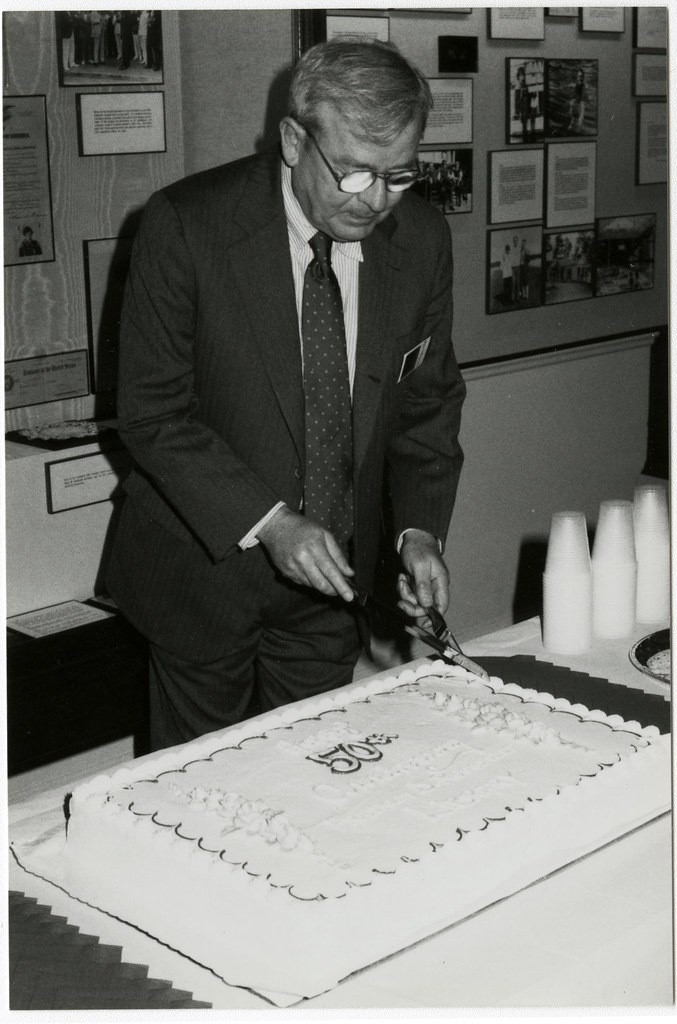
[307,130,428,195]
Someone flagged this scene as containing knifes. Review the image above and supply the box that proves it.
[344,576,490,682]
[406,575,463,667]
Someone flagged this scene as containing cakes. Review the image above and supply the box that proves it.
[66,657,673,959]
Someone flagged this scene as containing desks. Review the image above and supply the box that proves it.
[8,598,677,1010]
[8,596,151,780]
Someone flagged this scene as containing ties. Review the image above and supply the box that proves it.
[302,229,355,560]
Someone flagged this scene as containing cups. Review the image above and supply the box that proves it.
[541,484,671,655]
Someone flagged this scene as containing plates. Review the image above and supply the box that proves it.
[628,628,671,690]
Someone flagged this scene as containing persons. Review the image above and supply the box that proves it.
[93,33,467,757]
[568,69,588,133]
[412,159,468,213]
[59,11,159,70]
[509,72,538,142]
[500,236,532,302]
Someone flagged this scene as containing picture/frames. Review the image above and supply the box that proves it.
[321,6,668,316]
[55,10,165,88]
